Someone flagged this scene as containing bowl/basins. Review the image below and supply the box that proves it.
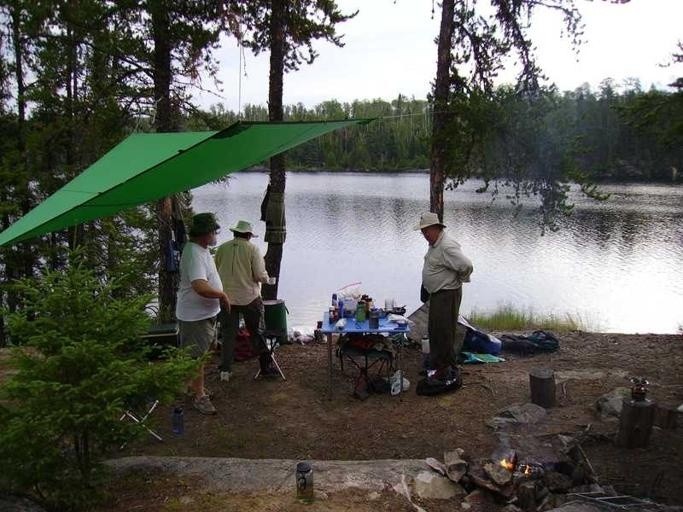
[393,307,406,315]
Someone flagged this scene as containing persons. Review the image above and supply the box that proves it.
[213,219,281,380]
[412,211,475,382]
[173,211,233,416]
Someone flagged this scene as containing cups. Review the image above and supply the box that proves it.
[385,298,394,313]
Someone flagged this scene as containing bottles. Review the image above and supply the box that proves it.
[296,462,313,506]
[173,407,184,435]
[317,321,323,329]
[328,293,379,329]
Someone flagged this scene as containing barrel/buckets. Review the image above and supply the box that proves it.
[264,299,288,344]
[620,399,656,449]
[529,368,556,406]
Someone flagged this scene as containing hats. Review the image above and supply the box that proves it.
[189,212,223,237]
[228,219,260,239]
[410,212,447,232]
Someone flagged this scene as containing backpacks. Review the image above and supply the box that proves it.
[500,329,559,356]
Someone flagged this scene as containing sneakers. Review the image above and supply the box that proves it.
[192,392,218,416]
[219,369,235,382]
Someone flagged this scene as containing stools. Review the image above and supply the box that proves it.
[251,328,285,381]
